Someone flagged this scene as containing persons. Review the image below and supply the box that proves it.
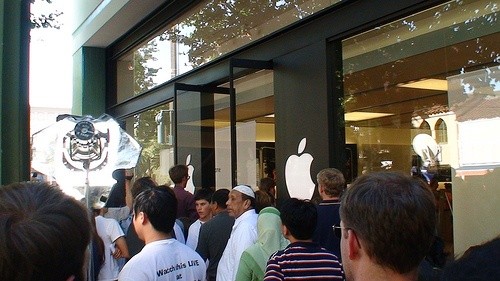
[90,169,207,281]
[234,207,291,281]
[186,187,236,281]
[333,169,438,281]
[169,164,200,223]
[313,167,346,253]
[215,184,259,281]
[0,181,90,281]
[263,199,346,281]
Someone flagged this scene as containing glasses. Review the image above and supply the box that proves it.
[187,176,190,180]
[333,225,361,249]
